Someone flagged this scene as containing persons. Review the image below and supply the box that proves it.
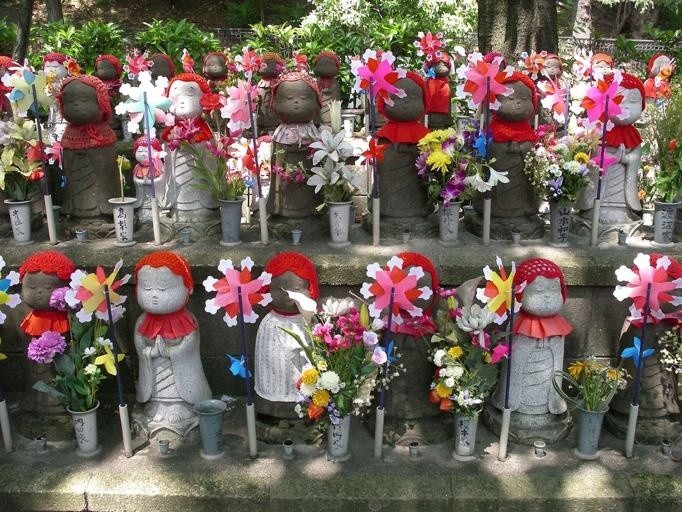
[366,252,453,419]
[490,258,574,418]
[254,252,320,418]
[19,250,75,418]
[130,251,213,419]
[611,253,682,419]
[1,53,681,244]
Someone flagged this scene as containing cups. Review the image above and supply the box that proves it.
[291,229,302,243]
[533,440,545,456]
[408,441,420,458]
[182,230,193,243]
[34,435,48,452]
[660,438,671,456]
[399,230,411,243]
[342,114,356,140]
[282,438,295,458]
[76,230,86,242]
[618,232,628,245]
[510,229,521,244]
[158,439,171,456]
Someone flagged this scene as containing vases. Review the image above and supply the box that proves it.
[66,402,102,451]
[192,401,227,453]
[577,406,607,454]
[453,408,480,456]
[329,412,349,459]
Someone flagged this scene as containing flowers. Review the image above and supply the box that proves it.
[0,31,681,424]
